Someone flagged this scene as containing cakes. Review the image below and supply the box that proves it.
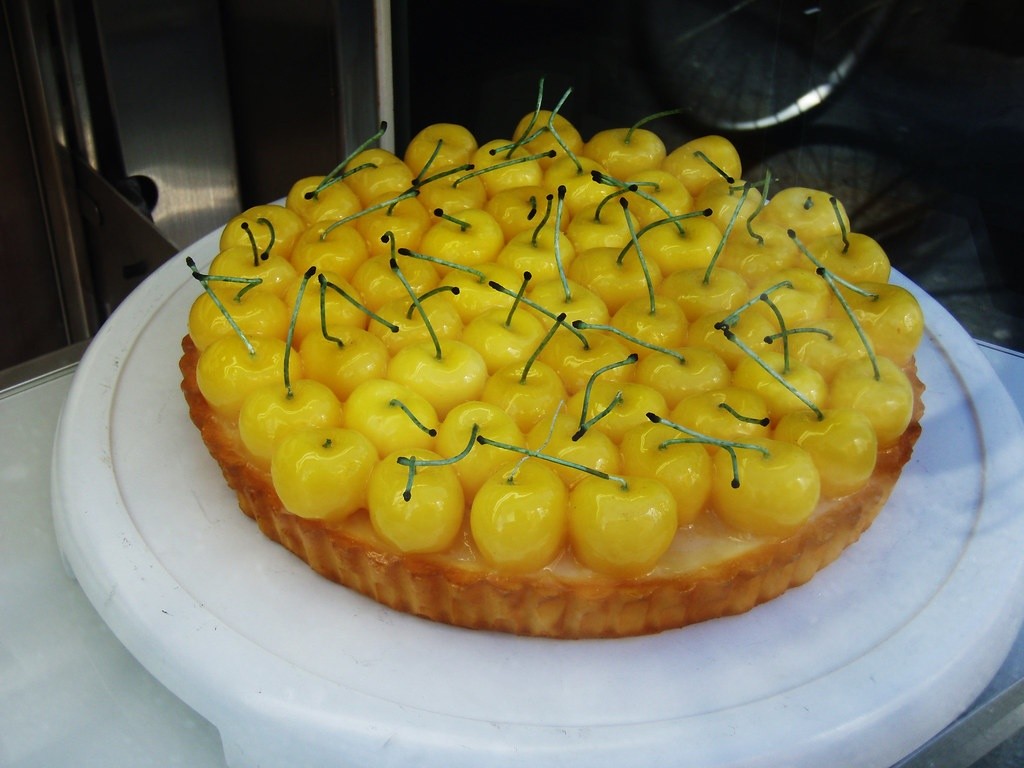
[176,80,925,636]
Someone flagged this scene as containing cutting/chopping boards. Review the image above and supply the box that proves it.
[47,194,1021,768]
